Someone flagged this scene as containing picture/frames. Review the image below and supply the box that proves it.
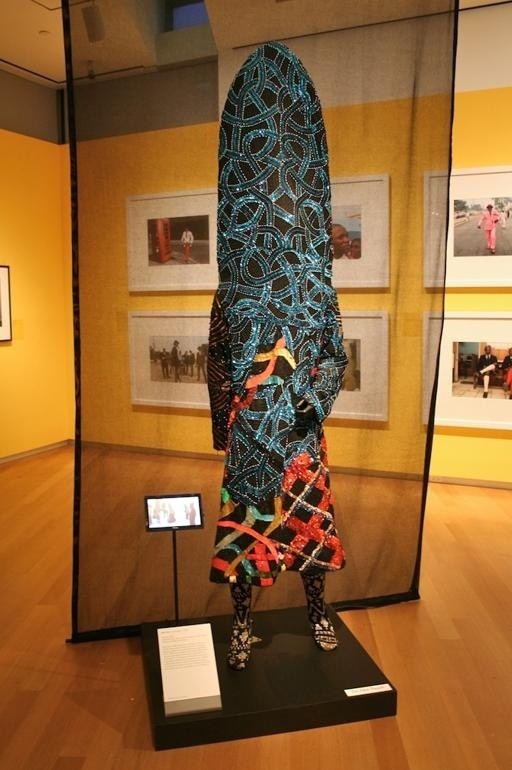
[328,173,392,289]
[420,311,512,431]
[315,311,389,422]
[126,187,224,292]
[422,166,512,287]
[128,310,213,410]
[0,265,12,342]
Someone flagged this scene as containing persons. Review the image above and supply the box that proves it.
[498,210,507,229]
[501,348,512,399]
[158,338,209,385]
[177,226,195,264]
[347,235,362,260]
[475,203,502,254]
[476,345,498,398]
[332,223,349,262]
[203,332,352,672]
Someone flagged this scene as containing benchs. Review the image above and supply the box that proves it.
[473,360,505,389]
